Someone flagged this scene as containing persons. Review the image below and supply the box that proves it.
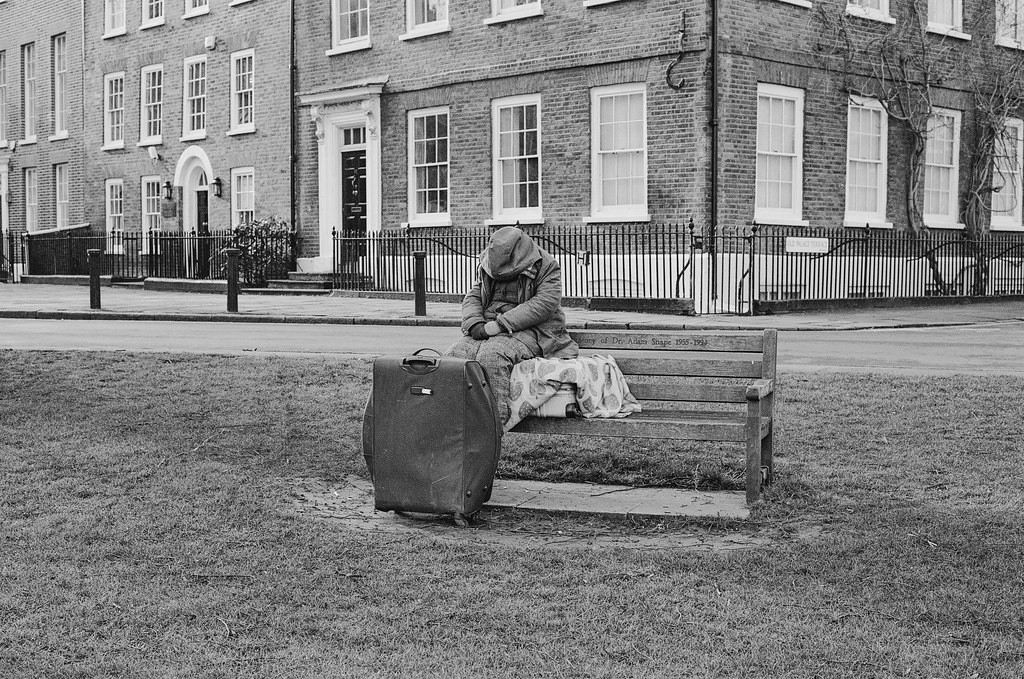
[441,227,579,427]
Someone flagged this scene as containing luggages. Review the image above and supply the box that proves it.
[363,347,501,527]
[526,382,584,419]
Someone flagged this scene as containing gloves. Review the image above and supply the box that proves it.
[470,322,490,340]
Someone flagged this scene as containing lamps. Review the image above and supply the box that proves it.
[6,189,12,203]
[211,178,221,197]
[162,181,172,199]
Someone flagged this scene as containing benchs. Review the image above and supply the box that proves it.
[508,328,778,504]
